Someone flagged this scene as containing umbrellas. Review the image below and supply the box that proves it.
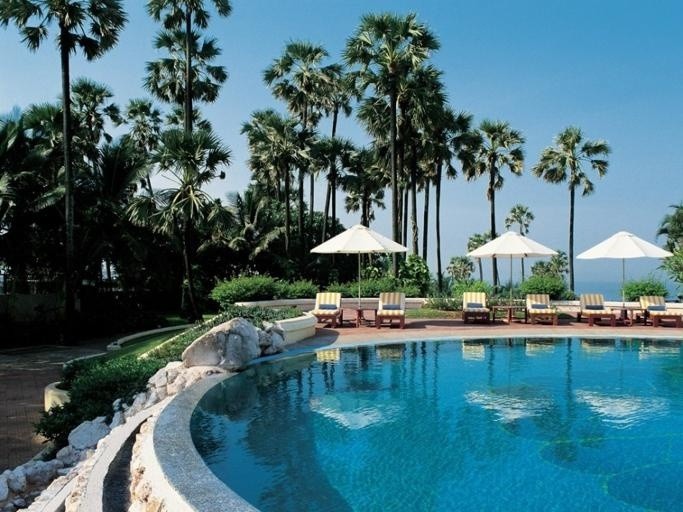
[308,351,408,430]
[572,350,670,431]
[466,231,560,321]
[309,224,409,324]
[464,348,555,424]
[576,231,675,308]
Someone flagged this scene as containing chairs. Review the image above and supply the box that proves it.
[577,294,616,327]
[462,292,490,325]
[636,296,682,328]
[525,294,558,326]
[311,292,344,329]
[313,335,683,364]
[377,292,406,330]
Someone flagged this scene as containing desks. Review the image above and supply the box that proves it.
[611,308,647,327]
[356,304,377,328]
[493,306,513,325]
